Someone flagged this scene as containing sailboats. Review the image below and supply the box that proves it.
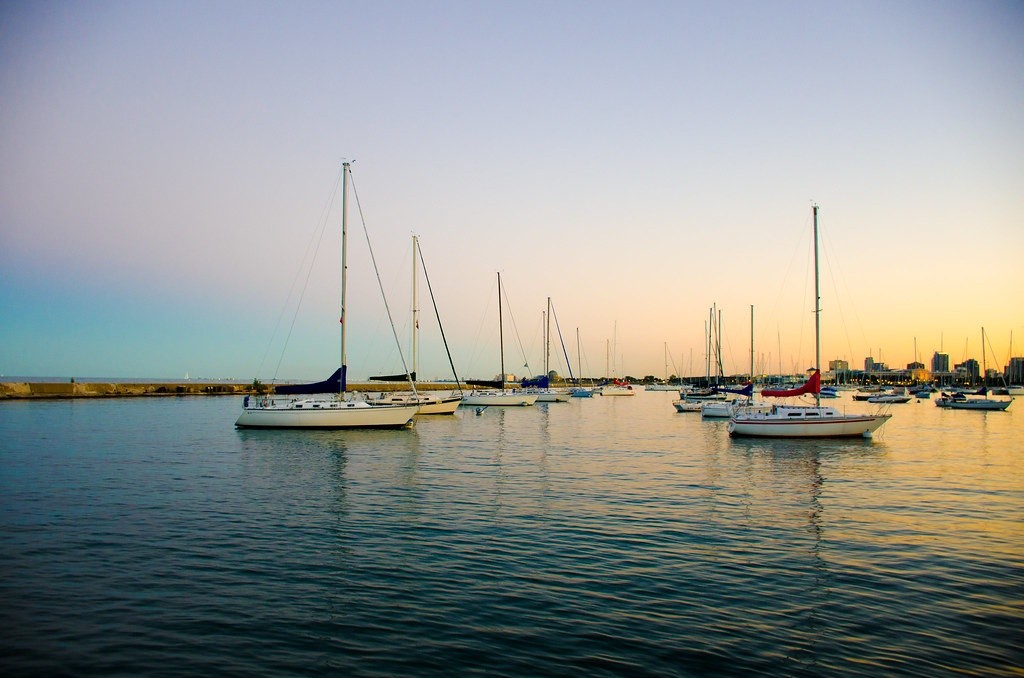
[452,267,541,407]
[934,328,1019,415]
[525,293,1024,418]
[234,154,420,429]
[724,207,895,439]
[367,235,464,413]
